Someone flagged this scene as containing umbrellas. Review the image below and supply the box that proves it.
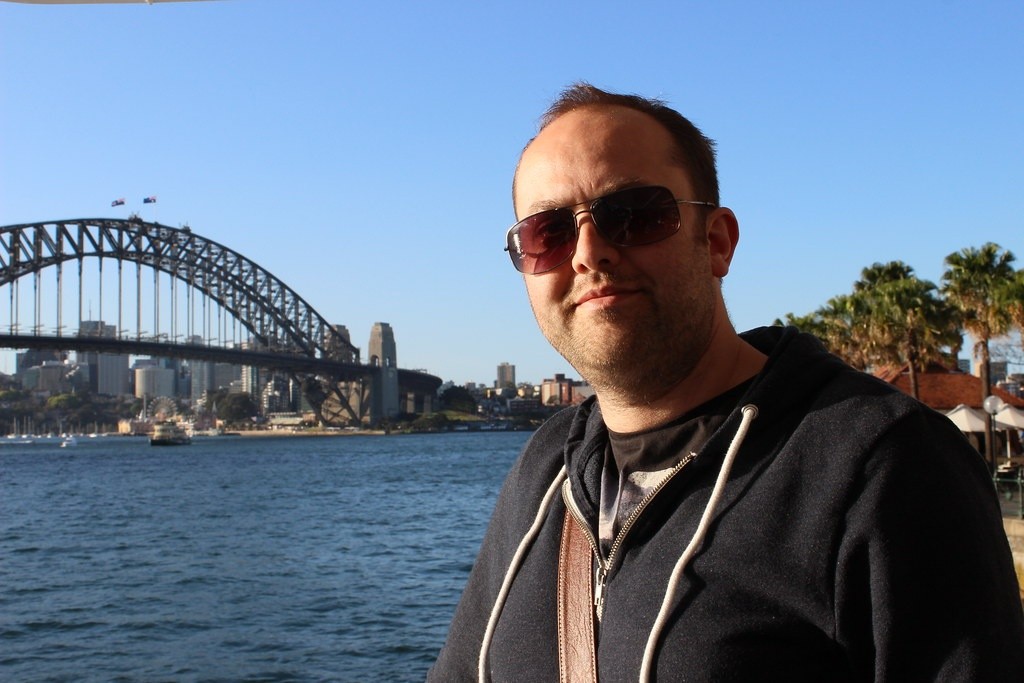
[944,403,1006,432]
[992,406,1023,467]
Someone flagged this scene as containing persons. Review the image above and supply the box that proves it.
[418,79,1023,683]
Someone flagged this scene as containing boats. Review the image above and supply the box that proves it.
[149,418,193,447]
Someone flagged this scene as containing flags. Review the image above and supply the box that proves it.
[143,195,157,204]
[111,198,125,207]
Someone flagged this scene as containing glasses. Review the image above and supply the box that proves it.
[504,184,719,275]
